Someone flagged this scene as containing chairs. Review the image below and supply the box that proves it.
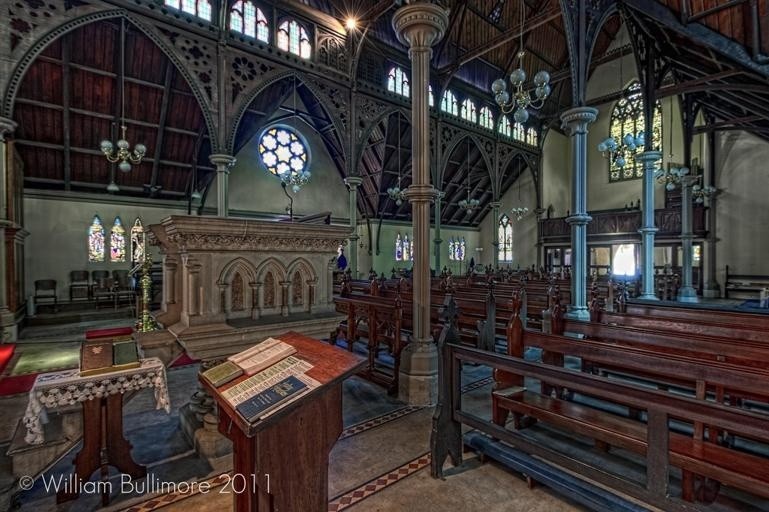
[34,268,136,312]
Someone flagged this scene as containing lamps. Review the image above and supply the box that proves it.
[100,18,147,173]
[388,0,717,223]
[276,75,315,192]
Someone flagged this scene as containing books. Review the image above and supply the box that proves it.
[227,337,296,377]
[202,361,243,388]
[235,375,309,422]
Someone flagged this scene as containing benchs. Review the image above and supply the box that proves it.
[332,265,768,512]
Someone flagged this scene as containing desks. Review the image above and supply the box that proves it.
[30,357,163,505]
